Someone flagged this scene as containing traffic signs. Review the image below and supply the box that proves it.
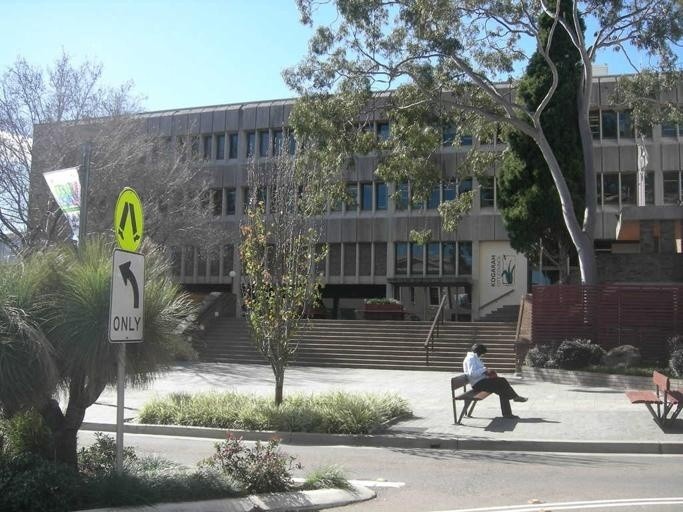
[108,247,149,343]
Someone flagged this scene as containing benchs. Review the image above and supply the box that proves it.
[451,374,491,424]
[653,370,683,430]
[625,370,668,430]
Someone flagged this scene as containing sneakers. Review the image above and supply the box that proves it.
[514,396,528,401]
[503,414,518,418]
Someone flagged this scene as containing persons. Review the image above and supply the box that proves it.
[463,344,528,418]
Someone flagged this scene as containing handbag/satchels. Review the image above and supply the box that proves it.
[483,371,497,379]
